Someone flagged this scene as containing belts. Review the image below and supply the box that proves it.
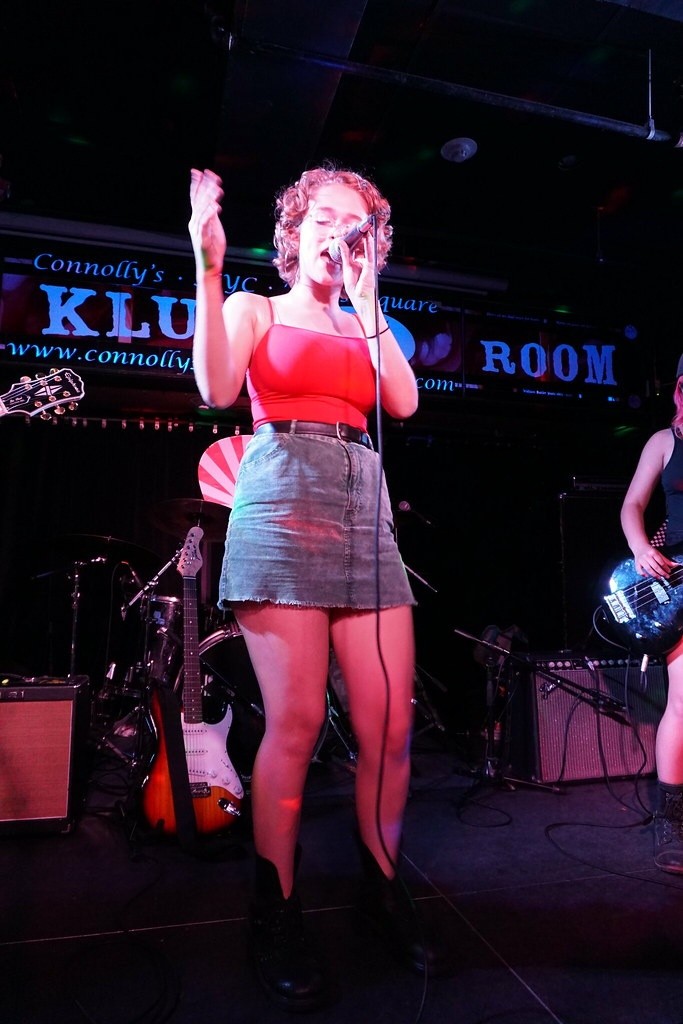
[254,420,372,449]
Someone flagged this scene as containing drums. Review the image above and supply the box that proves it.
[102,657,148,700]
[173,621,334,797]
[145,596,185,684]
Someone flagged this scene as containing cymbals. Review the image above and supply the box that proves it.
[144,497,231,544]
[61,531,161,562]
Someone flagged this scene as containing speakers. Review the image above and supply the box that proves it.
[552,491,668,655]
[0,684,82,832]
[508,652,669,787]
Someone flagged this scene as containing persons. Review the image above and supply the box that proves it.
[189,166,449,1006]
[621,354,683,873]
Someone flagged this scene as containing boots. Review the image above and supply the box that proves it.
[350,823,428,985]
[250,841,318,998]
[655,778,683,874]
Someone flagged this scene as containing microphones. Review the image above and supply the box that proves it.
[598,699,634,713]
[329,215,377,265]
[125,564,144,591]
[398,501,431,525]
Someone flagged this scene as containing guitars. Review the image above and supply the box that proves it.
[599,552,682,655]
[142,527,246,837]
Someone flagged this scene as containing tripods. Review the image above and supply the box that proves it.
[444,627,633,808]
[87,519,201,780]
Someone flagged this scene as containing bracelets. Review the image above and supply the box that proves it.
[363,324,390,339]
